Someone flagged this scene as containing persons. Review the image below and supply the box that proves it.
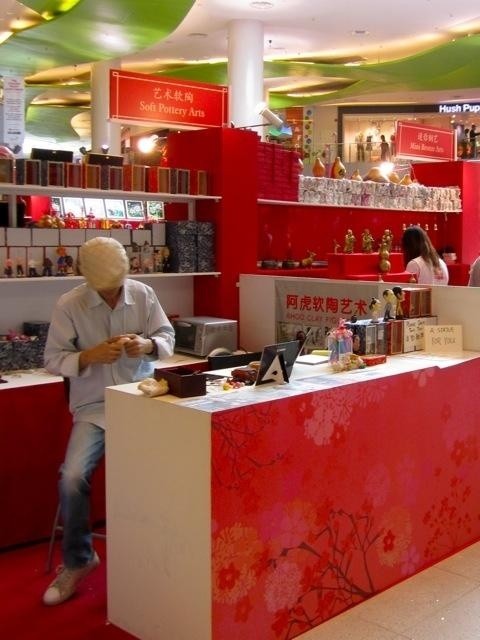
[366,130,374,162]
[469,124,480,160]
[381,287,395,321]
[343,228,356,253]
[41,236,177,605]
[353,130,365,162]
[369,297,381,323]
[392,287,406,320]
[390,131,396,157]
[362,228,376,254]
[461,128,469,159]
[377,135,391,162]
[400,224,450,285]
[378,229,395,254]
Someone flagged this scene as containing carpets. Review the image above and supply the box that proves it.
[2,520,151,639]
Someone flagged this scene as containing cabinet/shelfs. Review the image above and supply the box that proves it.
[0,187,225,390]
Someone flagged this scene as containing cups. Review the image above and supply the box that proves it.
[256,260,328,269]
[442,252,457,264]
[298,176,461,212]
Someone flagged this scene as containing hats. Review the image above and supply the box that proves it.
[77,236,130,291]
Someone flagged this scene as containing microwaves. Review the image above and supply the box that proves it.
[170,316,238,357]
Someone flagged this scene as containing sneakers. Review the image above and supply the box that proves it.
[40,550,102,607]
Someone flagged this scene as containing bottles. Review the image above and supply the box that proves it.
[312,156,414,185]
[38,209,133,230]
[127,240,170,273]
